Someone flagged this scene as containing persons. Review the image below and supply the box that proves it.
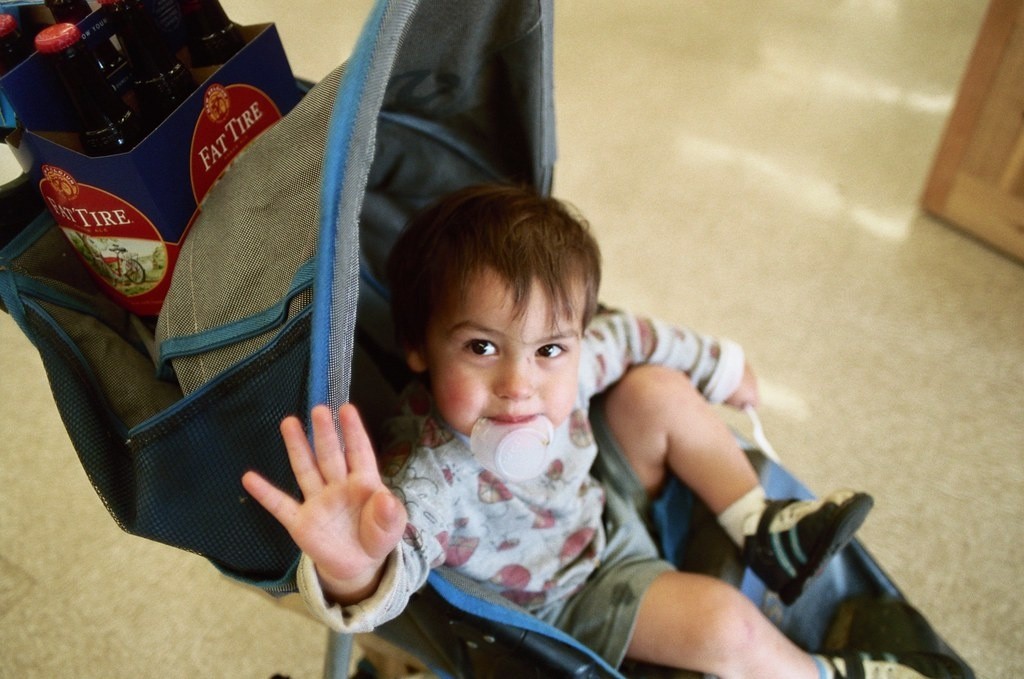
[243,185,873,679]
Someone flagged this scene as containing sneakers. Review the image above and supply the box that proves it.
[814,646,966,679]
[743,489,873,607]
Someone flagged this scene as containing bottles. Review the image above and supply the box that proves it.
[0,0,246,157]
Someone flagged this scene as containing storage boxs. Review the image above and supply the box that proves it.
[0,0,304,316]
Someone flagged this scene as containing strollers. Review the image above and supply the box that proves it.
[2,1,977,679]
